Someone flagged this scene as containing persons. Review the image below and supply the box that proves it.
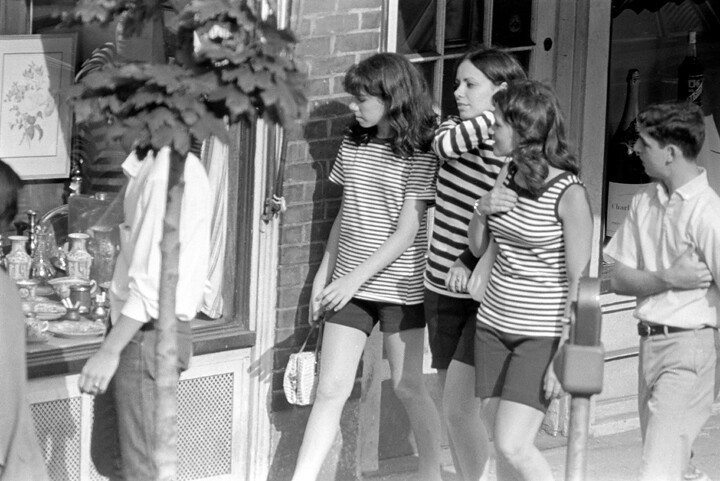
[468,80,593,481]
[290,53,442,481]
[422,47,528,481]
[603,100,720,481]
[77,129,213,481]
[0,159,51,481]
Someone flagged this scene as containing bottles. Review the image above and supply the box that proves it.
[2,227,116,320]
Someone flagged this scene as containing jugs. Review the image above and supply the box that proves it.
[49,277,96,300]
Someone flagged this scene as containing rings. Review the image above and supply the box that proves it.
[93,382,100,388]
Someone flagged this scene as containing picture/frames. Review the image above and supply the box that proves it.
[0,29,79,183]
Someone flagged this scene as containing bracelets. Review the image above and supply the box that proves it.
[473,199,485,216]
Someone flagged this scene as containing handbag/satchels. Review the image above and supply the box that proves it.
[283,316,324,406]
[467,232,499,303]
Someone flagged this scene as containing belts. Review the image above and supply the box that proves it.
[638,323,718,337]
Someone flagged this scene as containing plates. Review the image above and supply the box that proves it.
[48,320,106,339]
[34,303,67,319]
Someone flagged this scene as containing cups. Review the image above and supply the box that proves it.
[25,313,50,342]
